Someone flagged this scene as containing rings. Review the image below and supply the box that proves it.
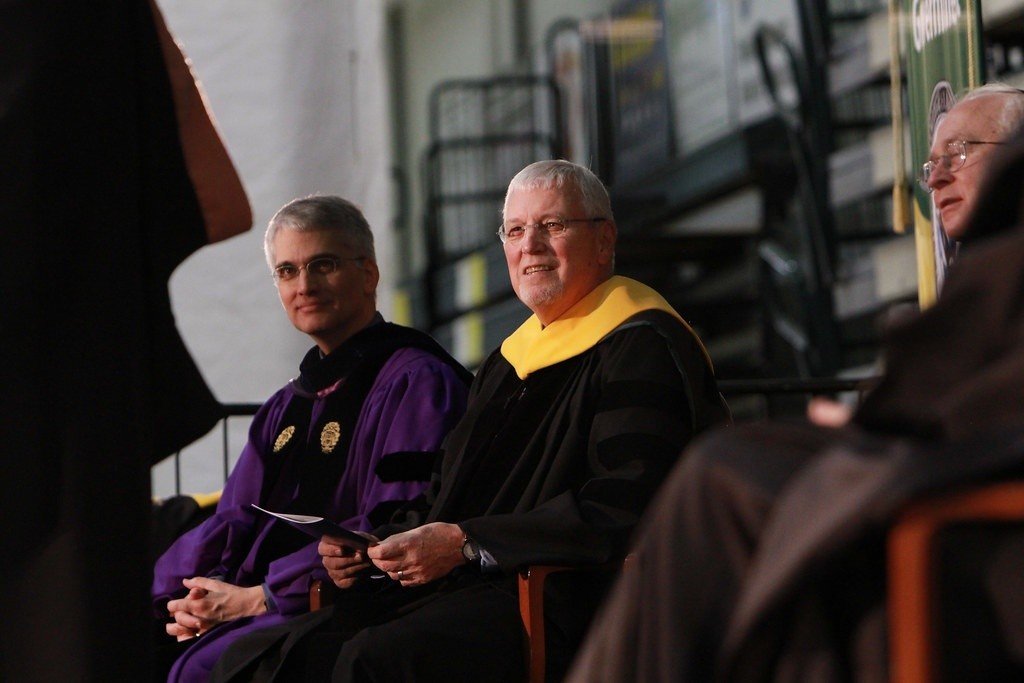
[192,630,198,637]
[398,570,406,580]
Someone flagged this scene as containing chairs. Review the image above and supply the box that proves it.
[311,0,1024,683]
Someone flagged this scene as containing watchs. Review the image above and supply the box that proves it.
[461,534,480,562]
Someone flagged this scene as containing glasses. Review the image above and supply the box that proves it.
[916,140,1010,194]
[273,256,363,282]
[495,217,604,244]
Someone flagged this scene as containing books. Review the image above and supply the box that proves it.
[252,504,379,557]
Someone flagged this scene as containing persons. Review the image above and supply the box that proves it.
[0,0,252,683]
[209,159,722,682]
[565,85,1024,683]
[150,197,474,683]
